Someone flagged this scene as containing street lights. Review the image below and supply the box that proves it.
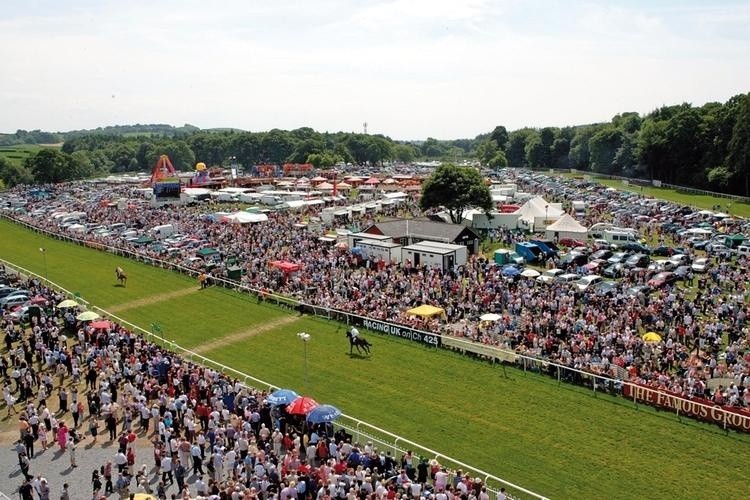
[298,332,312,398]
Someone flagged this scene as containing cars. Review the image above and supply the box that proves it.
[501,167,750,308]
[0,271,49,324]
[333,161,517,184]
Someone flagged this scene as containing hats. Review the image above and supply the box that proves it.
[289,481,296,488]
[430,459,438,466]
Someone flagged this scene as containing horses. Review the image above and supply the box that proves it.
[115,268,128,285]
[345,331,372,355]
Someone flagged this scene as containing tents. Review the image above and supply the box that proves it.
[405,305,449,324]
[183,182,590,242]
[132,235,154,247]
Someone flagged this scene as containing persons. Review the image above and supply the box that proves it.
[117,266,123,277]
[2,182,539,344]
[198,272,207,289]
[350,326,359,342]
[1,263,749,500]
[518,182,747,262]
